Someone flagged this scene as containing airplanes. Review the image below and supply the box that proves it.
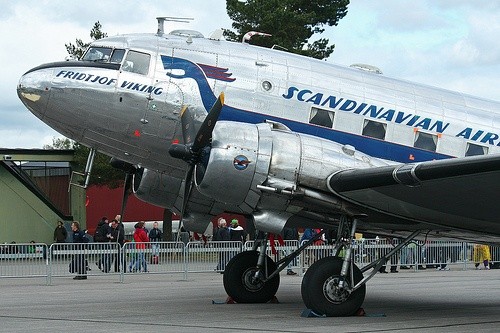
[17,16,500,316]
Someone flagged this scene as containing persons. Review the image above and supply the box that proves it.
[128,231,143,273]
[213,217,223,272]
[131,223,150,272]
[114,215,127,273]
[180,226,190,242]
[227,218,247,261]
[71,221,88,280]
[284,227,499,275]
[215,218,231,274]
[148,221,163,264]
[81,229,92,271]
[139,220,149,236]
[0,240,46,262]
[116,56,133,73]
[102,219,122,274]
[53,221,68,261]
[93,217,111,271]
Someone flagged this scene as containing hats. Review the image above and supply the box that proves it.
[230,219,237,223]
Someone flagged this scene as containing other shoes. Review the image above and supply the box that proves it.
[379,264,490,272]
[287,270,297,275]
